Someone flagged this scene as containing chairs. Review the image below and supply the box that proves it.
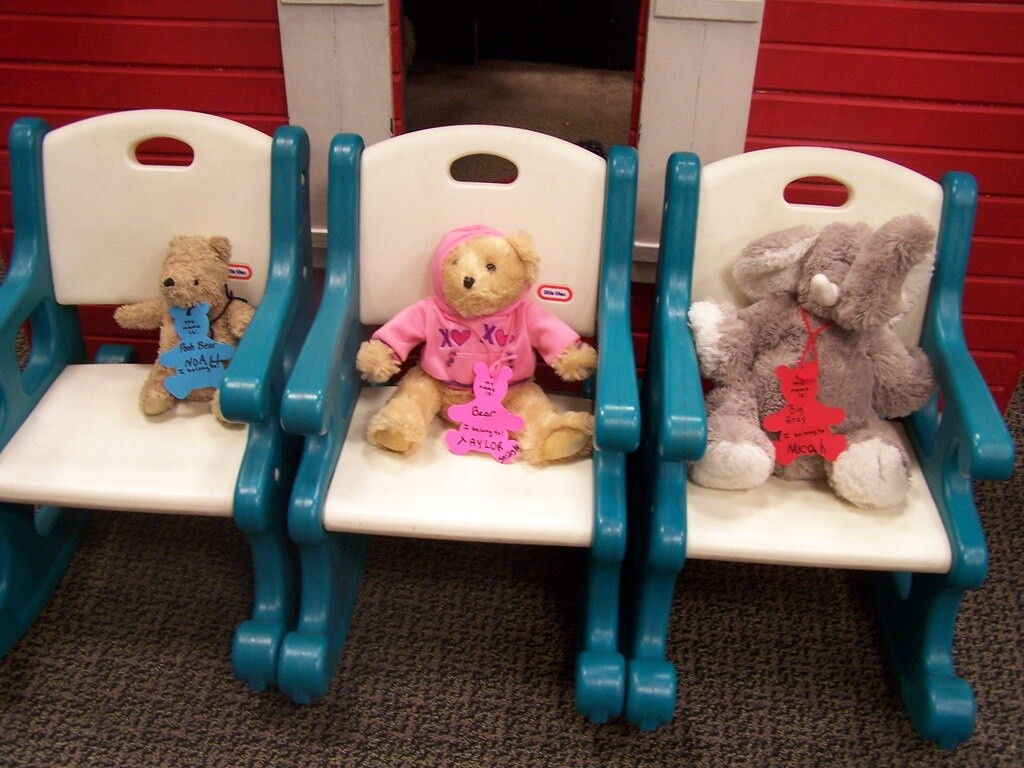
[275,120,642,725]
[0,108,316,692]
[624,145,1016,752]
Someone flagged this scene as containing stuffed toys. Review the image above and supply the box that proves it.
[113,235,257,424]
[356,222,596,467]
[687,211,935,512]
